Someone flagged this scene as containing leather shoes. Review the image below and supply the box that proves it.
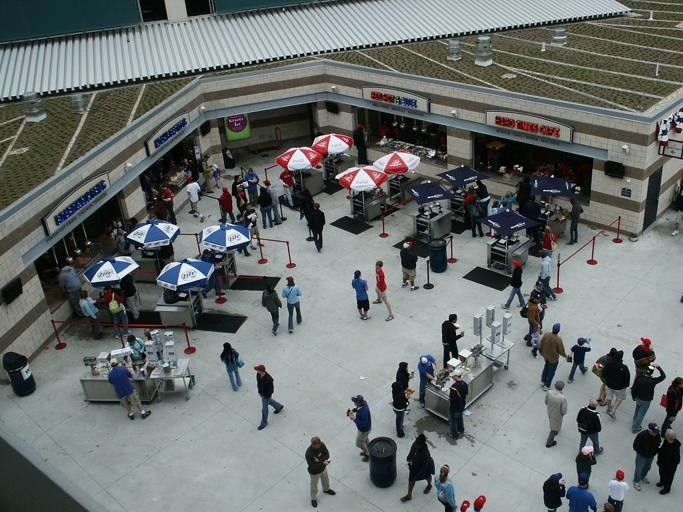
[400,496,412,501]
[423,485,432,494]
[656,482,663,487]
[659,488,669,494]
[323,488,335,495]
[258,422,267,430]
[274,405,284,414]
[545,441,556,448]
[312,500,317,507]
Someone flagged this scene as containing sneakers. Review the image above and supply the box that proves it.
[402,282,408,287]
[515,304,524,308]
[128,413,135,420]
[386,316,394,321]
[258,241,264,247]
[124,329,131,337]
[116,335,119,339]
[360,315,363,319]
[363,315,371,320]
[360,451,365,456]
[410,286,419,290]
[373,300,382,304]
[608,411,617,419]
[641,479,649,484]
[250,245,257,250]
[607,406,612,412]
[362,455,369,462]
[632,481,640,491]
[501,304,509,309]
[598,447,602,452]
[142,411,151,419]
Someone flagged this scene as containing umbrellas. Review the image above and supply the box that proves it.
[84,255,141,287]
[372,151,421,183]
[127,218,180,274]
[156,257,219,328]
[436,165,485,188]
[484,212,539,239]
[312,133,353,183]
[275,146,324,191]
[531,175,573,212]
[406,182,453,206]
[334,165,388,225]
[199,223,253,255]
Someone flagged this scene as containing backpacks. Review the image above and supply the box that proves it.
[163,287,179,305]
[108,292,122,314]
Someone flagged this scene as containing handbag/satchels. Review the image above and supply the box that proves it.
[439,490,447,504]
[234,351,244,368]
[660,387,679,410]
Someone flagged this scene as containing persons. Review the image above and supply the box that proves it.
[632,337,657,376]
[201,154,214,193]
[576,399,604,454]
[530,330,541,358]
[143,174,153,198]
[278,167,296,209]
[124,335,146,362]
[474,178,490,217]
[231,175,250,216]
[391,380,413,439]
[353,126,369,165]
[542,473,566,512]
[120,274,140,320]
[575,446,597,477]
[630,363,667,434]
[473,495,487,512]
[346,395,372,462]
[418,354,439,409]
[107,358,152,420]
[503,191,515,208]
[441,314,464,369]
[434,465,457,512]
[244,203,264,250]
[304,436,337,508]
[128,216,138,227]
[400,239,420,291]
[215,251,227,275]
[567,337,592,384]
[502,200,509,209]
[671,177,683,237]
[541,224,558,257]
[566,198,584,246]
[544,381,567,448]
[660,377,683,438]
[523,281,547,341]
[526,293,548,347]
[187,160,200,183]
[516,174,533,208]
[151,186,159,198]
[212,164,221,188]
[468,196,486,237]
[488,201,501,239]
[280,276,303,335]
[110,220,126,240]
[253,365,284,430]
[566,474,598,512]
[236,214,252,257]
[396,361,416,415]
[102,284,131,340]
[116,227,131,256]
[307,201,326,252]
[300,187,315,227]
[502,260,526,310]
[521,195,542,234]
[592,348,617,407]
[261,281,282,337]
[160,184,177,225]
[538,250,557,301]
[219,186,236,224]
[463,185,476,209]
[159,241,174,265]
[219,342,242,392]
[656,429,681,495]
[257,186,273,229]
[608,469,630,512]
[185,175,206,223]
[245,167,260,206]
[78,289,105,340]
[373,260,394,321]
[263,180,283,226]
[448,371,469,441]
[633,423,662,491]
[55,259,83,320]
[539,323,570,391]
[351,270,370,320]
[399,433,436,503]
[154,198,168,222]
[605,350,630,419]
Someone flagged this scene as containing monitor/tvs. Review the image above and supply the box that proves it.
[604,161,624,179]
[0,277,23,305]
[200,120,211,136]
[326,102,338,113]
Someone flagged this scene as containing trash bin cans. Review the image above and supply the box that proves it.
[3,352,36,397]
[427,238,447,273]
[368,437,397,487]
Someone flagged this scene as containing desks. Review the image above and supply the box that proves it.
[130,251,174,284]
[153,287,204,328]
[347,191,387,222]
[487,235,536,274]
[413,208,452,241]
[215,252,238,290]
[538,215,566,238]
[387,173,422,206]
[451,189,465,223]
[79,360,161,404]
[91,288,132,319]
[423,354,493,426]
[294,169,323,197]
[150,358,192,401]
[471,336,515,370]
[323,156,358,183]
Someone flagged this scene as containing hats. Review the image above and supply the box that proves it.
[582,446,593,455]
[553,323,560,332]
[254,365,265,372]
[578,472,588,480]
[641,337,651,345]
[352,395,363,402]
[649,423,660,432]
[110,358,118,365]
[264,180,270,183]
[474,495,486,509]
[615,470,624,480]
[512,259,521,267]
[549,473,562,481]
[223,186,228,192]
[421,357,428,364]
